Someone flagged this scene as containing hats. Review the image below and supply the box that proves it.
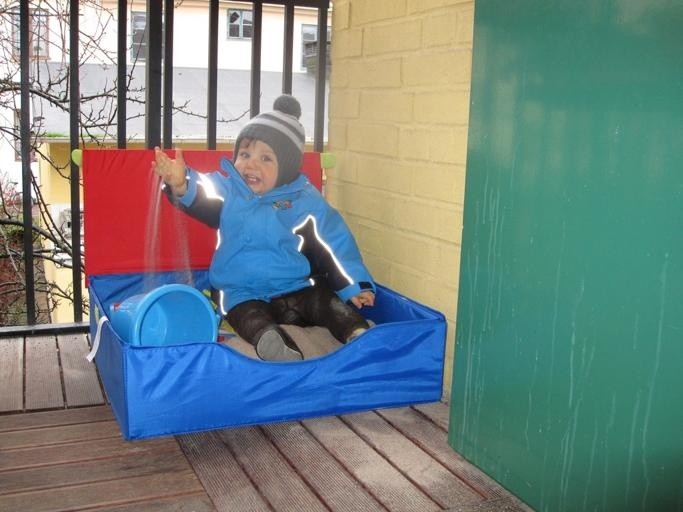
[233,94,304,189]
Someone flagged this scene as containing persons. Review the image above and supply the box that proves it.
[151,94,376,363]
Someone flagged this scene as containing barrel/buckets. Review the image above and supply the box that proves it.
[112,283,218,348]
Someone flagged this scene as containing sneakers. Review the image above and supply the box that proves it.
[254,327,304,362]
